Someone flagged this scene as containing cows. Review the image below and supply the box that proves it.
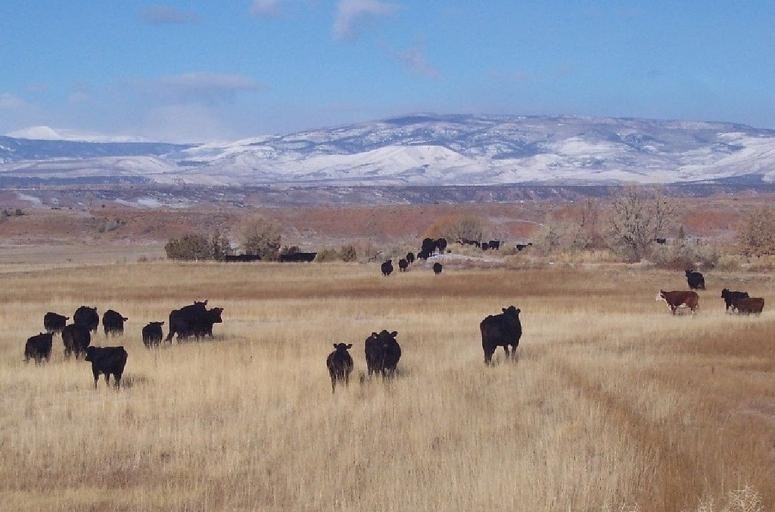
[43,306,128,359]
[721,288,765,315]
[85,345,128,389]
[23,332,53,366]
[480,305,522,366]
[327,342,353,394]
[456,237,500,250]
[381,260,393,277]
[656,289,699,316]
[685,269,706,290]
[365,330,401,379]
[142,300,224,349]
[515,243,532,251]
[399,238,447,274]
